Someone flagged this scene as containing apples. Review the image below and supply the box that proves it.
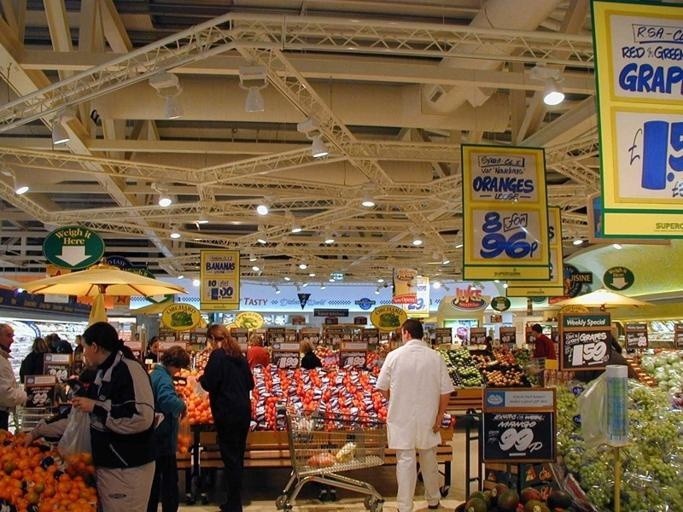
[473,350,525,386]
[251,363,388,431]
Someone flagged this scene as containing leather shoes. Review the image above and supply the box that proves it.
[218,503,243,512]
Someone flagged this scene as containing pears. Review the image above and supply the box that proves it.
[439,349,483,387]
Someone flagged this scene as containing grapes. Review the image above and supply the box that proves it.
[555,379,683,512]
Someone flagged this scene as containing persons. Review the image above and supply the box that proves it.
[482,336,496,361]
[15,331,84,408]
[373,319,455,511]
[142,335,159,374]
[118,339,136,361]
[573,321,640,382]
[530,323,557,388]
[300,339,323,369]
[199,323,256,511]
[247,333,271,369]
[0,323,35,432]
[22,321,159,512]
[146,345,191,512]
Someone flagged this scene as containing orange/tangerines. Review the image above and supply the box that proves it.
[0,428,98,512]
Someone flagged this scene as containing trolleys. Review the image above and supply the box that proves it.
[271,388,390,512]
[13,405,48,436]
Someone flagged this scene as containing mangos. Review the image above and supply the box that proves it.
[464,483,577,512]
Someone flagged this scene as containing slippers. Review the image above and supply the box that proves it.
[428,502,440,510]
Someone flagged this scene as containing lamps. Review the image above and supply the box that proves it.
[378,277,384,283]
[168,223,182,239]
[320,282,325,289]
[299,262,307,270]
[13,174,29,195]
[273,285,281,293]
[413,235,422,245]
[165,95,183,119]
[433,281,441,289]
[456,238,463,248]
[284,277,291,281]
[49,109,71,145]
[571,231,583,247]
[245,87,265,112]
[152,183,172,207]
[531,61,567,108]
[329,273,336,283]
[324,231,334,244]
[257,234,266,243]
[302,281,307,287]
[291,224,302,232]
[177,273,184,279]
[257,199,275,215]
[306,128,329,157]
[362,193,375,207]
[309,271,316,277]
[249,249,257,261]
[252,264,260,271]
[193,279,200,287]
[197,208,208,224]
[442,255,450,265]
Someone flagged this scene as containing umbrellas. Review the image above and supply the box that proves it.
[550,282,654,310]
[20,256,189,299]
